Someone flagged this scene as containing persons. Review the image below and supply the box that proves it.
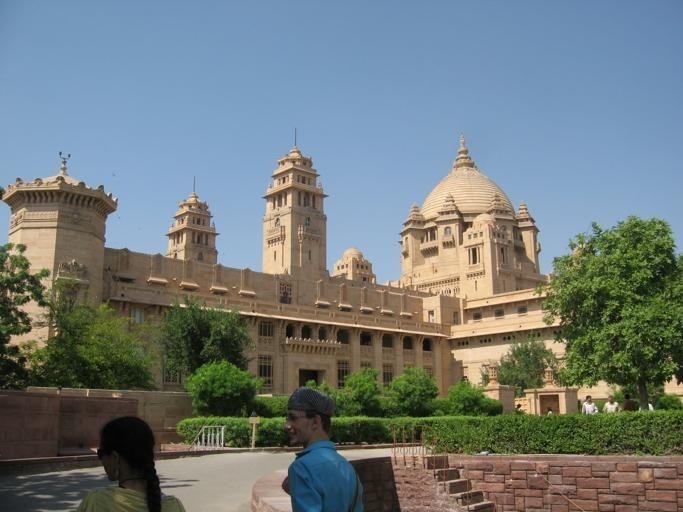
[603,396,621,413]
[638,401,653,412]
[620,393,636,412]
[516,404,522,411]
[582,396,598,414]
[285,388,366,512]
[547,407,552,415]
[79,416,185,512]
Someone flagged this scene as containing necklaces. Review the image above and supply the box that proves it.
[119,476,143,484]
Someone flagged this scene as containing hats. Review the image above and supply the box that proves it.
[287,387,333,416]
